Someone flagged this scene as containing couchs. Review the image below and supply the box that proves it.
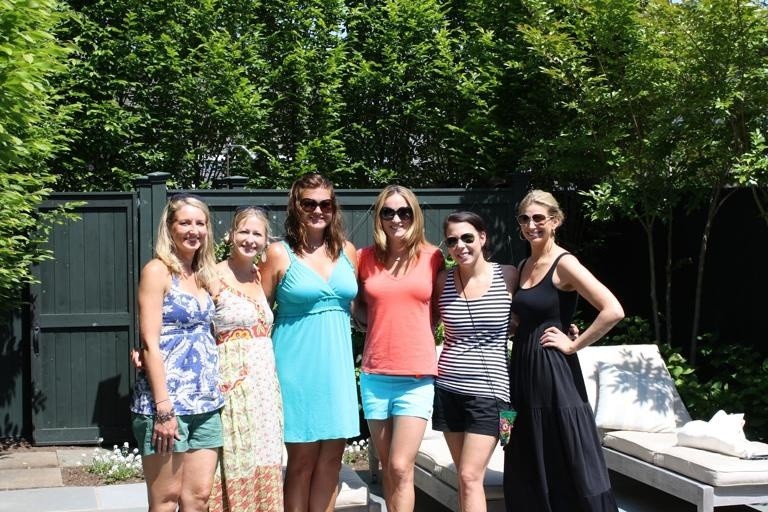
[577,343,767,512]
[282,346,505,512]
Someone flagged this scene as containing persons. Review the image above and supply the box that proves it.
[124,194,262,512]
[355,183,447,512]
[510,187,626,511]
[128,203,287,512]
[354,209,580,512]
[255,169,363,512]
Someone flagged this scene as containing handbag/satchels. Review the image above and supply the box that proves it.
[498,410,517,445]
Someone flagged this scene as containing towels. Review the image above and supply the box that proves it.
[677,410,767,459]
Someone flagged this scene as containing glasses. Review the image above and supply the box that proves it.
[380,206,413,220]
[517,213,555,225]
[445,233,474,247]
[300,198,336,214]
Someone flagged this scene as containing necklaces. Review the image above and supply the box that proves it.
[394,255,401,262]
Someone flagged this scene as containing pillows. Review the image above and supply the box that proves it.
[594,367,675,433]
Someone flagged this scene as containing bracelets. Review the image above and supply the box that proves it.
[152,408,176,425]
[153,398,171,406]
